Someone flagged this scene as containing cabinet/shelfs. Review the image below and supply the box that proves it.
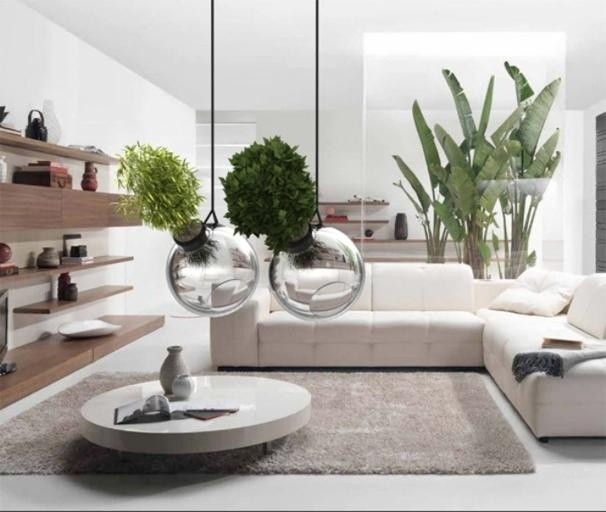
[0,128,144,230]
[0,253,165,410]
[309,199,514,244]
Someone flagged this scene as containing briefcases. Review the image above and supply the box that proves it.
[13,172,72,189]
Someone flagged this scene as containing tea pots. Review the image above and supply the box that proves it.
[24,107,47,141]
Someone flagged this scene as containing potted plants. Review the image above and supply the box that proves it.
[217,134,367,320]
[106,141,261,319]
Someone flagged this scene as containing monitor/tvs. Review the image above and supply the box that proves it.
[0,288,16,377]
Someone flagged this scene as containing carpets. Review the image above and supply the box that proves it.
[0,370,538,478]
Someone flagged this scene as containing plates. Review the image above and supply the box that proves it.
[56,319,121,338]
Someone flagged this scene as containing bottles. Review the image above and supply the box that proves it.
[1,155,8,185]
[395,213,408,240]
[35,247,61,269]
[57,272,71,298]
[172,374,195,399]
[68,282,78,300]
[80,161,98,191]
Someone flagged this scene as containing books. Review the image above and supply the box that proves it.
[114,393,239,425]
[60,255,93,265]
[541,336,583,350]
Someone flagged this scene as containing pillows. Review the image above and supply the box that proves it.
[567,273,606,340]
[488,267,575,317]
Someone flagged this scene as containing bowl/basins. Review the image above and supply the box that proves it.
[0,106,9,124]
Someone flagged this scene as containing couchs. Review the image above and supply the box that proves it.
[207,261,606,444]
